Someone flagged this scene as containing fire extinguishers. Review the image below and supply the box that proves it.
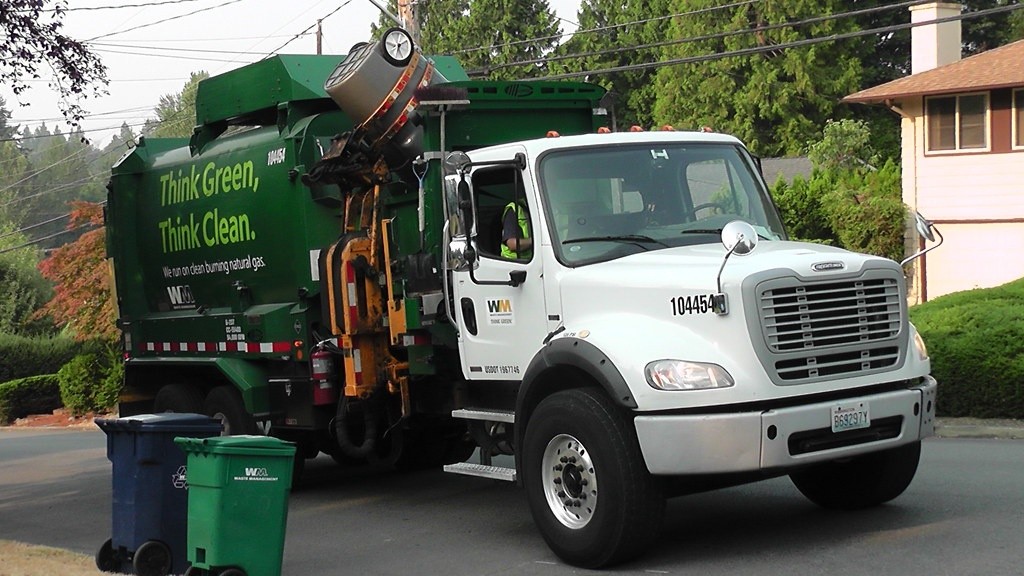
[308,338,338,406]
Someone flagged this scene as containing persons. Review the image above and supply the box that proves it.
[501,156,571,263]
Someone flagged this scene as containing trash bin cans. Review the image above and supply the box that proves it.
[173,433,295,576]
[325,27,451,160]
[94,412,225,576]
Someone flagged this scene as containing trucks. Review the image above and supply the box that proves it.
[102,26,944,567]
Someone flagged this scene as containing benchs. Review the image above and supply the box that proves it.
[478,205,670,254]
